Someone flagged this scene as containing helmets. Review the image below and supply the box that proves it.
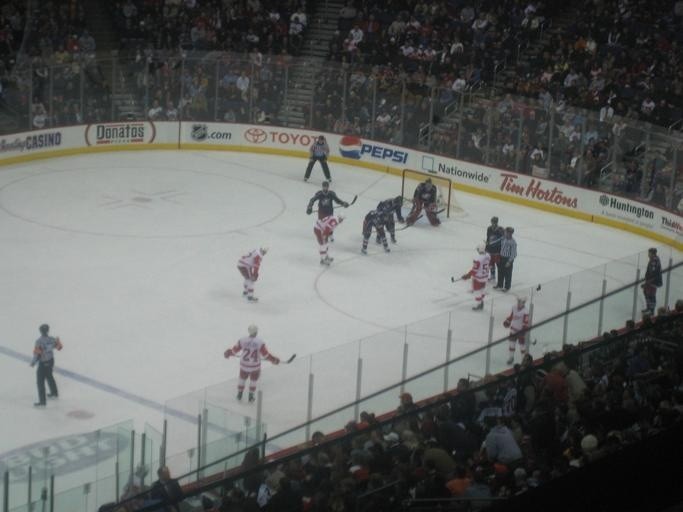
[476,242,485,253]
[247,324,258,335]
[517,295,527,304]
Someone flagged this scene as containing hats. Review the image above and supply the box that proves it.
[397,392,413,402]
[383,433,400,442]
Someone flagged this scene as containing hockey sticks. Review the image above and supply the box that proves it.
[231,355,296,365]
[403,204,445,214]
[371,223,411,232]
[451,277,462,282]
[313,195,357,212]
